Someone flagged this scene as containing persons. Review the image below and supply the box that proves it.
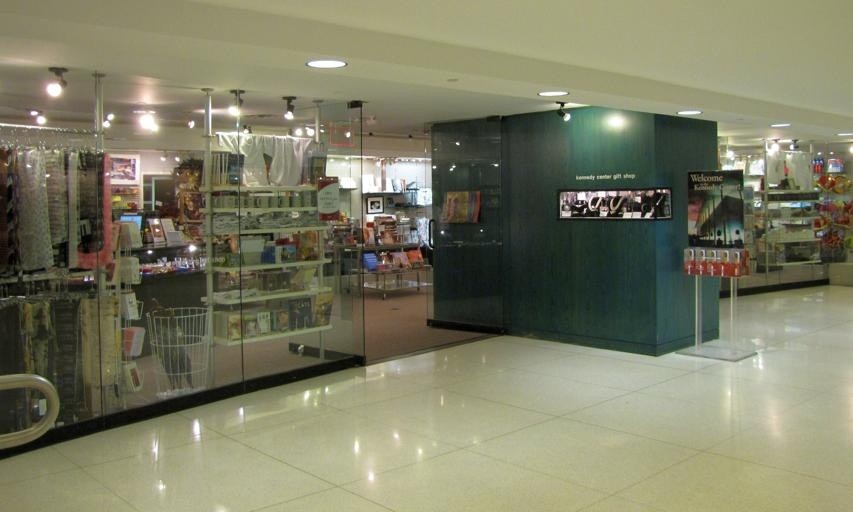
[643,190,666,217]
[688,196,703,235]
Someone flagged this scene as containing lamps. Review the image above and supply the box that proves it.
[556,99,573,122]
[790,140,799,150]
[43,65,69,100]
[226,87,245,117]
[282,94,298,122]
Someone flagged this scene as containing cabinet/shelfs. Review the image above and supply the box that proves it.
[768,228,822,267]
[212,137,336,349]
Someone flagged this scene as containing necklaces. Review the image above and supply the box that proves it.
[609,197,624,211]
[572,201,586,207]
[587,197,602,211]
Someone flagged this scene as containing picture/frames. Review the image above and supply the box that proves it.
[366,195,385,215]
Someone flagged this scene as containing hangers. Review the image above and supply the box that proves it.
[0,122,105,161]
[0,268,96,307]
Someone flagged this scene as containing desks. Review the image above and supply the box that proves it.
[347,262,433,302]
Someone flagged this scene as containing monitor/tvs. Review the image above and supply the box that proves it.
[119,215,146,234]
